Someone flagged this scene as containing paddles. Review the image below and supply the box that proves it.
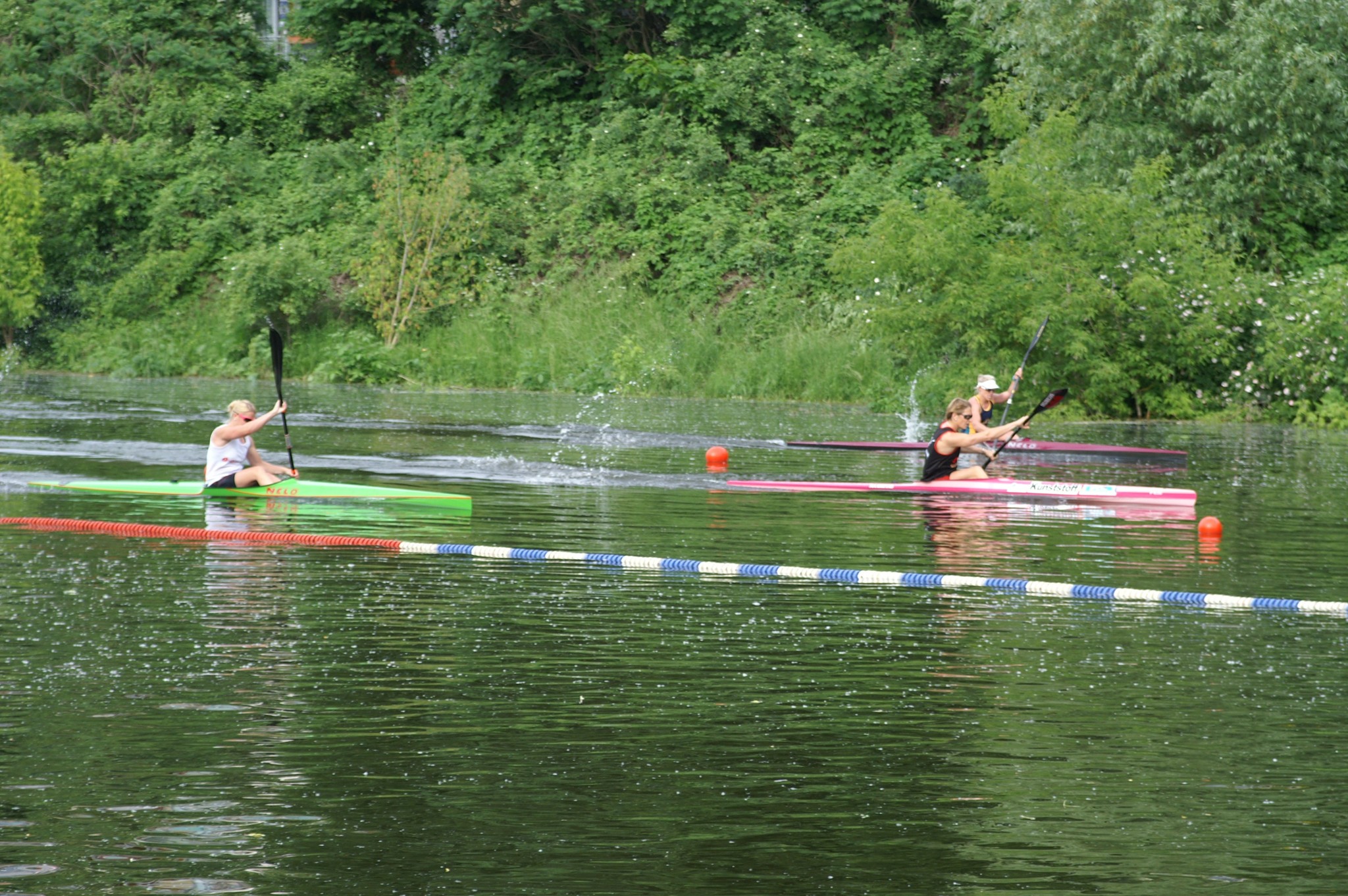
[268,311,296,474]
[992,314,1049,450]
[981,388,1068,470]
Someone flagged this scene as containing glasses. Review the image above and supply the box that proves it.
[986,388,995,392]
[960,413,972,420]
[238,413,254,422]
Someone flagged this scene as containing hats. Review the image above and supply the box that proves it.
[978,380,999,389]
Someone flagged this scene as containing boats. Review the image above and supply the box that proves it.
[725,478,1196,507]
[783,439,1182,456]
[39,471,472,512]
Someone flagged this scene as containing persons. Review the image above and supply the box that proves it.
[206,398,298,489]
[923,398,1031,482]
[967,368,1025,442]
